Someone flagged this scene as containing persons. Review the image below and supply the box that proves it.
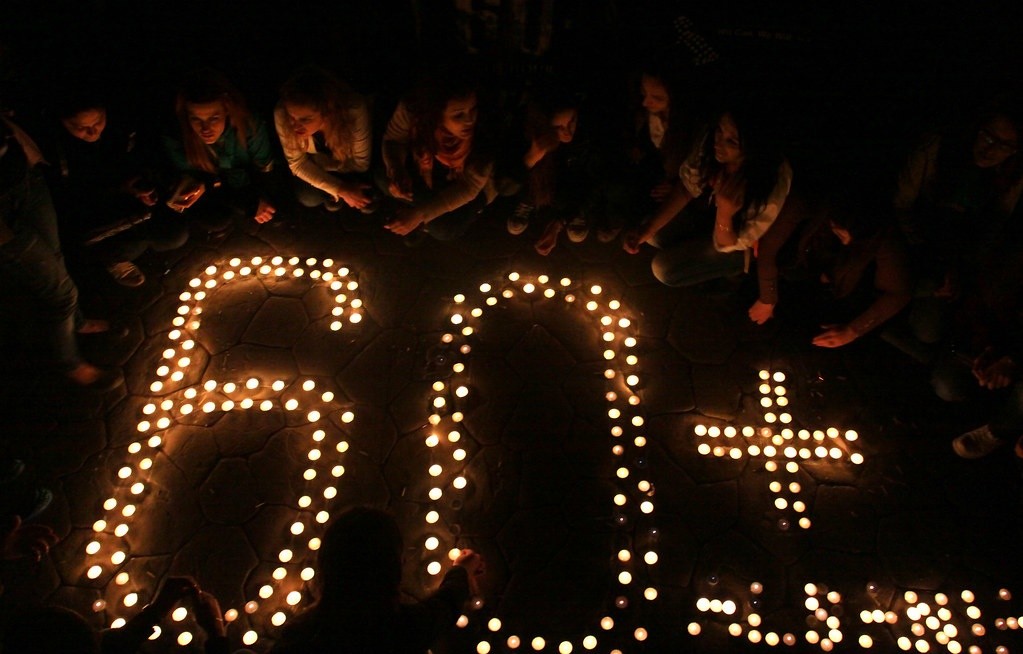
[1,47,1023,654]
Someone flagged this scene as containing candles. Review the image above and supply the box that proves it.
[83,256,1023,654]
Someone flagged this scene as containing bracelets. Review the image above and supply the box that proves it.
[716,220,732,233]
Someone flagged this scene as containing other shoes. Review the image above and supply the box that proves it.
[75,317,129,342]
[106,260,145,287]
[596,215,625,243]
[324,198,343,212]
[507,199,535,235]
[565,214,593,243]
[60,363,125,391]
[403,228,428,249]
[640,214,666,249]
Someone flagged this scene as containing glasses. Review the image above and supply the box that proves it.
[976,128,1018,154]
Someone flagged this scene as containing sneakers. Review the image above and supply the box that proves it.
[952,424,1002,459]
